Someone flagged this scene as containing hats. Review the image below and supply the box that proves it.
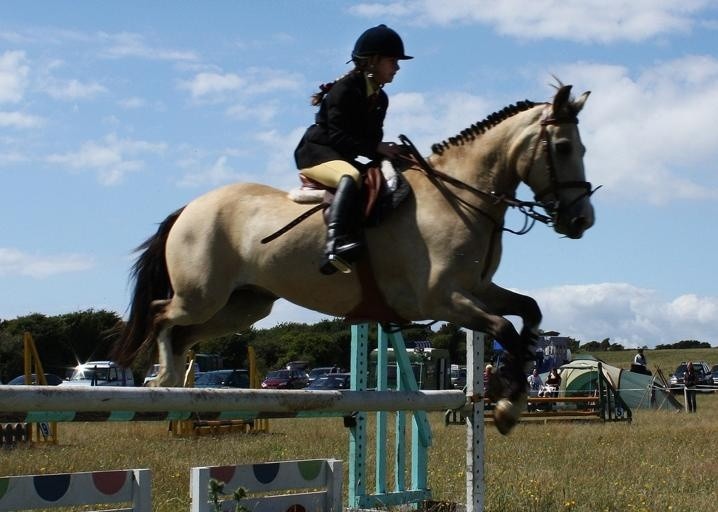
[350,24,415,62]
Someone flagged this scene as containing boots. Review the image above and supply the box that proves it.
[317,172,360,277]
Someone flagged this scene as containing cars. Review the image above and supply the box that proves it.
[142,361,350,389]
[710,364,718,384]
[669,362,712,388]
[8,370,60,386]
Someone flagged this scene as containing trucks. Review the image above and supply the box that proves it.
[531,336,575,375]
[369,345,454,390]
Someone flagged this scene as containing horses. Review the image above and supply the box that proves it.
[107,74,595,436]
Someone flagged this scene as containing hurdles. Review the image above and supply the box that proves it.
[1,331,60,445]
[170,346,268,437]
[444,361,633,426]
[0,326,484,512]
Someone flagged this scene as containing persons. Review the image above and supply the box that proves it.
[681,359,698,413]
[542,367,562,397]
[482,364,493,397]
[633,348,648,369]
[525,367,544,390]
[293,24,417,278]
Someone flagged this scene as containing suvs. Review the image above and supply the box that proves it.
[58,359,137,387]
[451,367,467,388]
